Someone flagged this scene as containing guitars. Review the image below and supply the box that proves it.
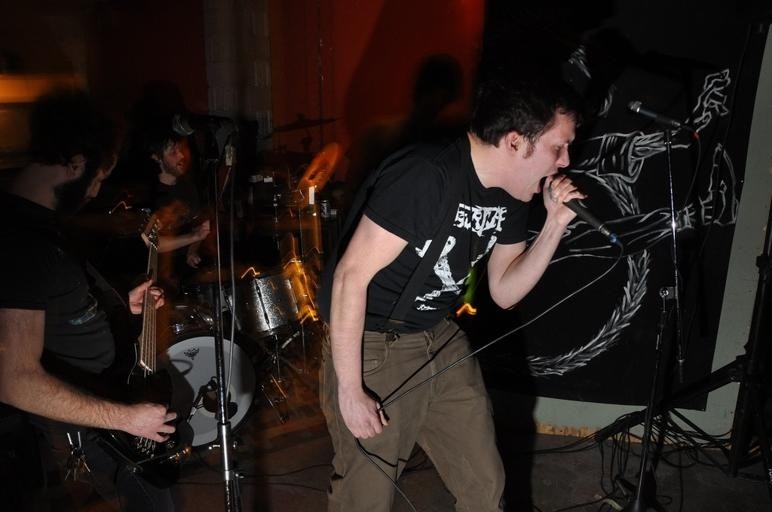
[96,209,195,487]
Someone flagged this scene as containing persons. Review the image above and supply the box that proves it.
[124,105,214,306]
[1,79,181,510]
[314,66,588,511]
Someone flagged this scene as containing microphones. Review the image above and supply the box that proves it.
[629,101,681,128]
[172,113,234,126]
[548,181,625,249]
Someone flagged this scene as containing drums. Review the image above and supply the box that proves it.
[228,265,299,337]
[137,304,256,447]
[194,264,234,312]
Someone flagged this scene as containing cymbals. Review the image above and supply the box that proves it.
[295,144,338,207]
[274,116,335,132]
